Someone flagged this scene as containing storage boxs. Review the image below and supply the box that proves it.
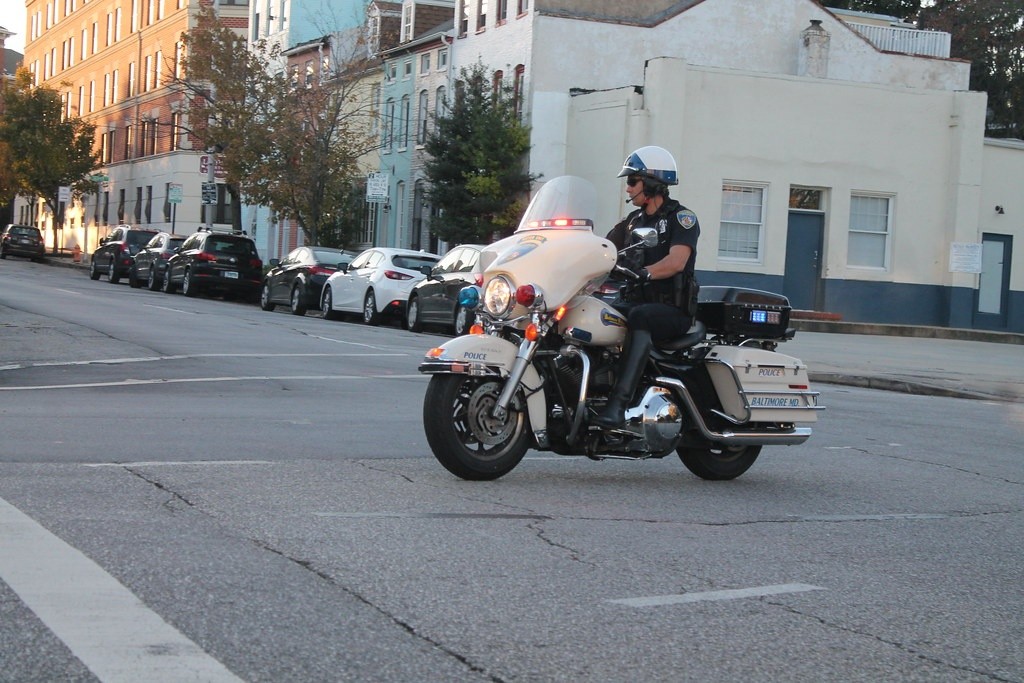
[698,284,796,338]
[706,341,814,422]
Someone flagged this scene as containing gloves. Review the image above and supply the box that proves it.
[623,266,650,302]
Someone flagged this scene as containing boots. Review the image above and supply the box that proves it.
[592,329,650,429]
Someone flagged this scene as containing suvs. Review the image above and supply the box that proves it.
[166,226,266,303]
[90,224,164,284]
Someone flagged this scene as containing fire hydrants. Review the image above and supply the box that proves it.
[72,244,83,263]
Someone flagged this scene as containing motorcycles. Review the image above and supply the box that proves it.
[415,172,826,480]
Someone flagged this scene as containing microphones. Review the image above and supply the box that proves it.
[626,190,643,203]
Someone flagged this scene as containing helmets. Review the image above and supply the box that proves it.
[617,146,678,185]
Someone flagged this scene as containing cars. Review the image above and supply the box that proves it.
[0,224,45,262]
[321,248,443,326]
[262,245,361,317]
[407,242,486,338]
[130,232,191,290]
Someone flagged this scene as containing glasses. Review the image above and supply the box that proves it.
[627,177,643,187]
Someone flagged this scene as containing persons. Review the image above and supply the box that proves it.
[588,145,701,429]
